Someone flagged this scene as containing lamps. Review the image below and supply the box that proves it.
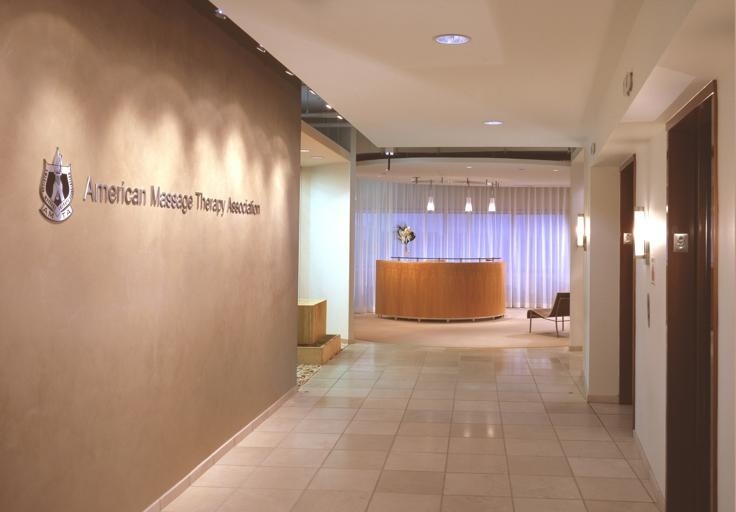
[213,9,343,120]
[632,206,646,258]
[488,183,497,212]
[426,180,435,212]
[575,214,585,248]
[464,182,472,212]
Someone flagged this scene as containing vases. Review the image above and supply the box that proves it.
[401,242,409,257]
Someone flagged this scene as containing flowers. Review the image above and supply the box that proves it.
[396,224,416,244]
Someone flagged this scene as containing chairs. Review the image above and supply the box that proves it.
[527,293,569,334]
